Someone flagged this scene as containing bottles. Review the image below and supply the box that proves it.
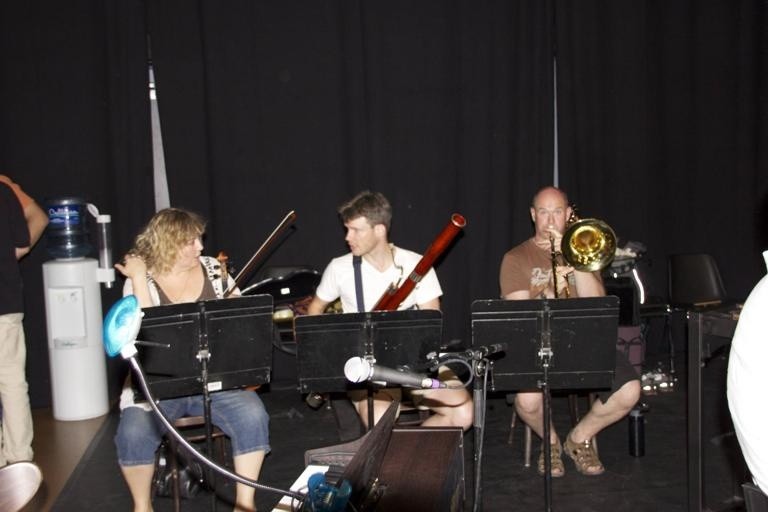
[86,202,117,290]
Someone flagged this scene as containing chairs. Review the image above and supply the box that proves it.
[394,403,433,428]
[506,392,599,477]
[159,416,232,511]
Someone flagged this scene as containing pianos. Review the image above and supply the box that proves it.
[269,396,467,512]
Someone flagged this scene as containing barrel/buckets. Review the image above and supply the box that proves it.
[46,195,89,259]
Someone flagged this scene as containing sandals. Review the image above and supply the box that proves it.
[564,430,605,476]
[536,437,565,478]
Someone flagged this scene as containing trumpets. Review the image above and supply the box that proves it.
[561,204,618,274]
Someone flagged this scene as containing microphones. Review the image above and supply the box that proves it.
[342,354,451,394]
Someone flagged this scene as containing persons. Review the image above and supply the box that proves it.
[0,170,51,469]
[499,187,641,477]
[113,206,272,512]
[307,191,474,435]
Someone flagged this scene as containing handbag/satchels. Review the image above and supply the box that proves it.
[152,442,206,506]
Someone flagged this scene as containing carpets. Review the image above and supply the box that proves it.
[51,355,753,512]
